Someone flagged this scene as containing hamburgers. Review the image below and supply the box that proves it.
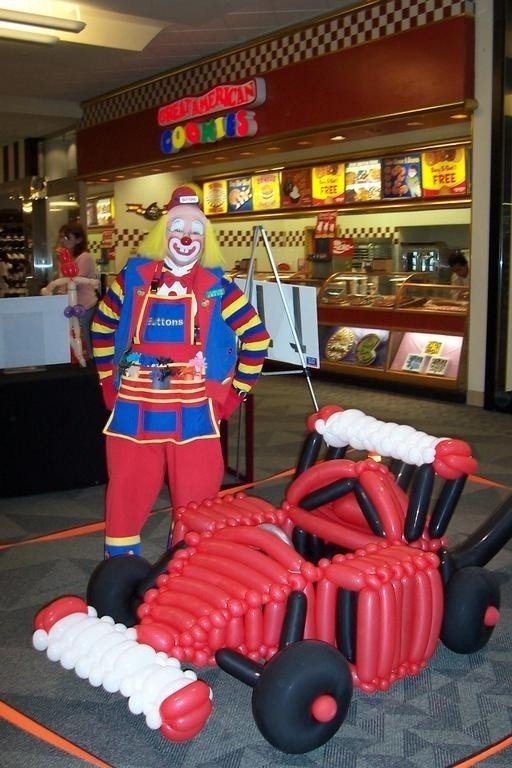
[261,184,273,199]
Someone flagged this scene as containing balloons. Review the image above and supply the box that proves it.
[40,247,101,369]
[31,400,511,755]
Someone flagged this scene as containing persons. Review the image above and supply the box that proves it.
[53,221,102,363]
[87,183,273,563]
[449,251,471,303]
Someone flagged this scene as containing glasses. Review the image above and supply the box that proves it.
[60,235,72,241]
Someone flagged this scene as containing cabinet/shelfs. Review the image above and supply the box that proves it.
[315,270,471,315]
[0,222,26,289]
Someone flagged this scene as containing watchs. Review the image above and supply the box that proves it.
[231,384,248,400]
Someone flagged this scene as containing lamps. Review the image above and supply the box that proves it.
[0,1,88,51]
